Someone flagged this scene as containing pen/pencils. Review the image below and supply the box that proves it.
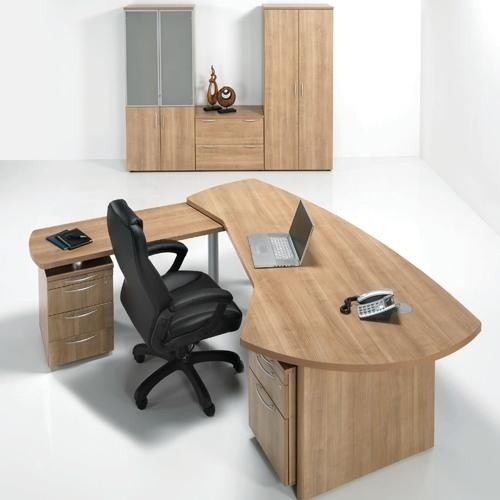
[66,235,88,239]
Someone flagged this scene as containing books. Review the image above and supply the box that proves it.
[57,227,93,250]
[46,229,69,250]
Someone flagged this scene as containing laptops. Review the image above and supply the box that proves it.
[246,199,315,269]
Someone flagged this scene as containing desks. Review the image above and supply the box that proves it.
[187,178,483,499]
[30,203,225,286]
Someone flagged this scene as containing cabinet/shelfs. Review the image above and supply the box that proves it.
[48,302,114,342]
[248,366,289,487]
[247,347,290,420]
[48,328,114,371]
[122,3,334,173]
[48,268,115,318]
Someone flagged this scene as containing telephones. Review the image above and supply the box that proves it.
[357,289,397,321]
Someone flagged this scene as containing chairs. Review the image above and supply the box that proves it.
[107,198,245,418]
